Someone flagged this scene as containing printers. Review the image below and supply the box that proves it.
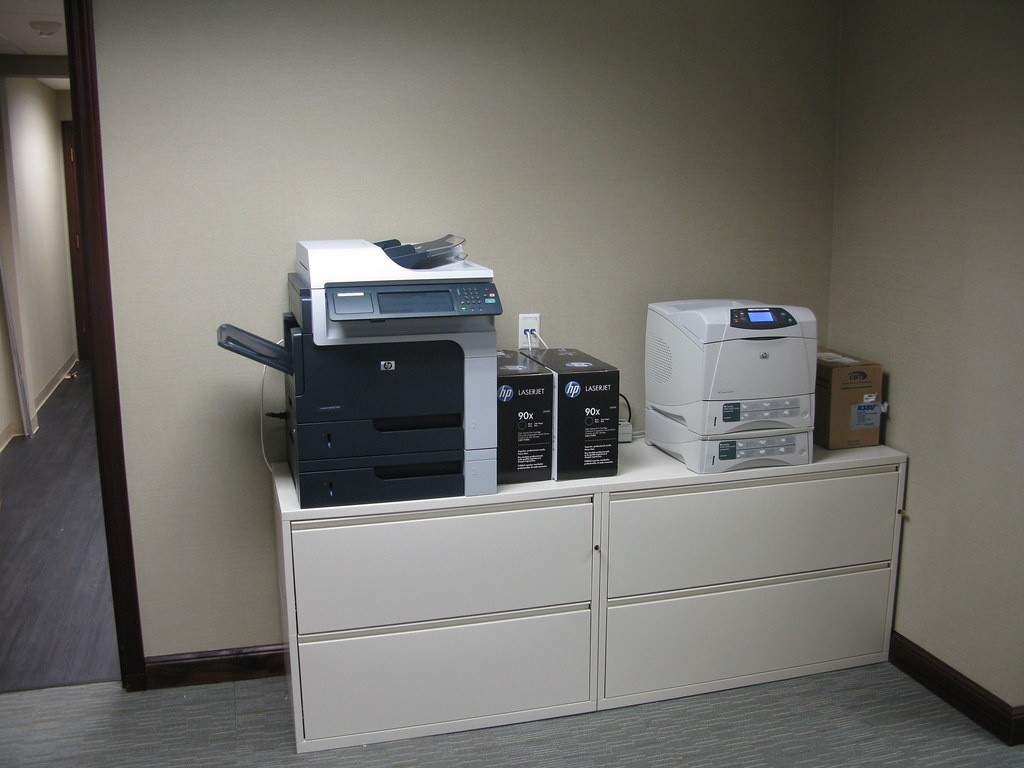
[645,298,816,475]
[216,233,503,510]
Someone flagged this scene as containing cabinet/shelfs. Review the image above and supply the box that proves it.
[268,440,908,755]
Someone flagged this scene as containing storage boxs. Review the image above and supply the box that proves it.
[497,350,553,485]
[814,349,882,450]
[519,348,620,482]
